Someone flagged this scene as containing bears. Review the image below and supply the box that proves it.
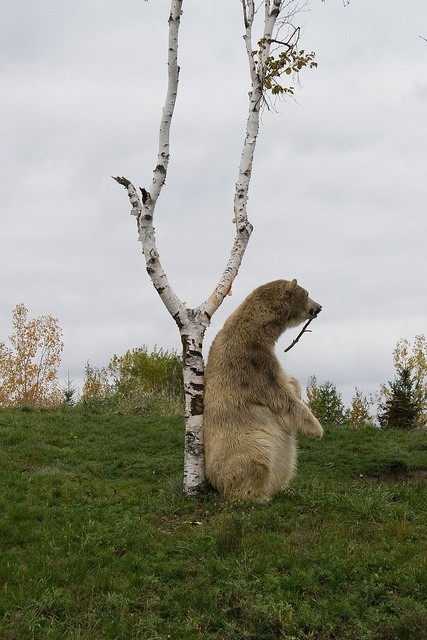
[204,279,325,503]
